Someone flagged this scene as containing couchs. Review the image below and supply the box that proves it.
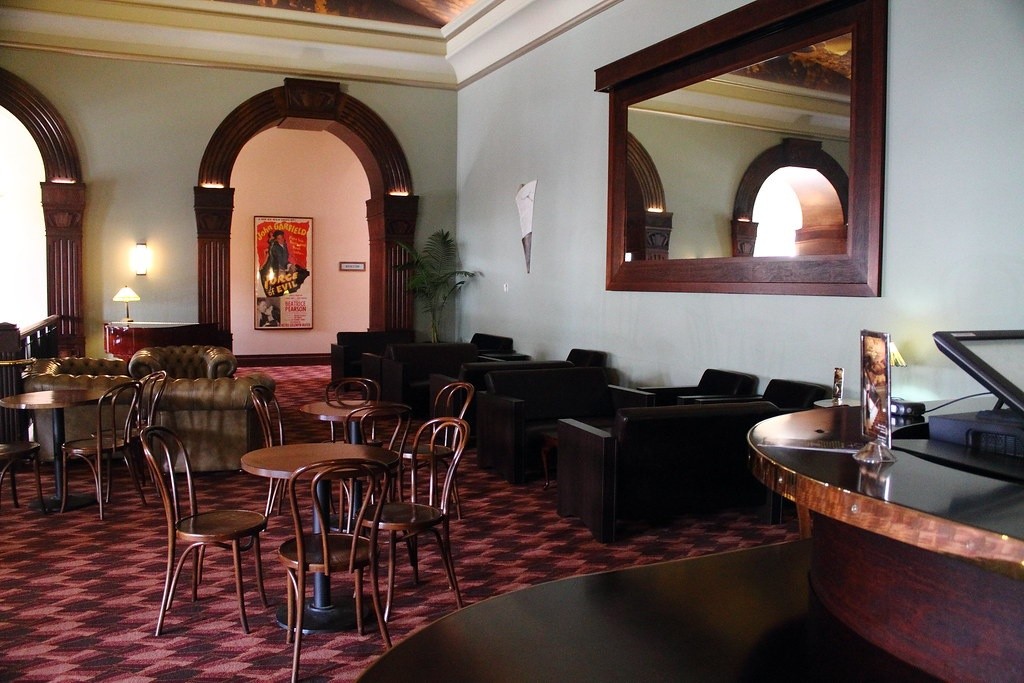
[21,356,141,466]
[138,371,276,477]
[128,345,237,378]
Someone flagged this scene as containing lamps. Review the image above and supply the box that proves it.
[112,285,140,322]
[135,243,147,275]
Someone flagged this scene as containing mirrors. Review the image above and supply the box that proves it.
[593,0,889,298]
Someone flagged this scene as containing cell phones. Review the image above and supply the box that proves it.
[890,397,925,416]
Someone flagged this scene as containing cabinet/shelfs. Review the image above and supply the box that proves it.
[104,322,218,362]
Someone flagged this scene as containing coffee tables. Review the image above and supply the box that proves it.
[541,427,613,490]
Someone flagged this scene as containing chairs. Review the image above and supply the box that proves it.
[58,381,146,521]
[89,370,168,503]
[138,424,267,638]
[392,382,474,519]
[325,377,384,505]
[249,384,337,531]
[0,442,43,507]
[277,459,392,683]
[337,403,410,537]
[352,416,470,622]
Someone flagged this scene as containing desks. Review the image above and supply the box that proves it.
[0,390,113,512]
[298,400,411,534]
[356,536,949,683]
[240,442,401,635]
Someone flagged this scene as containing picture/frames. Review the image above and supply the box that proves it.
[253,215,314,330]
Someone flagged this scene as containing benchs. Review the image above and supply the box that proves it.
[331,331,833,543]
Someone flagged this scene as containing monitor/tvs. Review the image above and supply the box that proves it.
[933,330,1024,418]
